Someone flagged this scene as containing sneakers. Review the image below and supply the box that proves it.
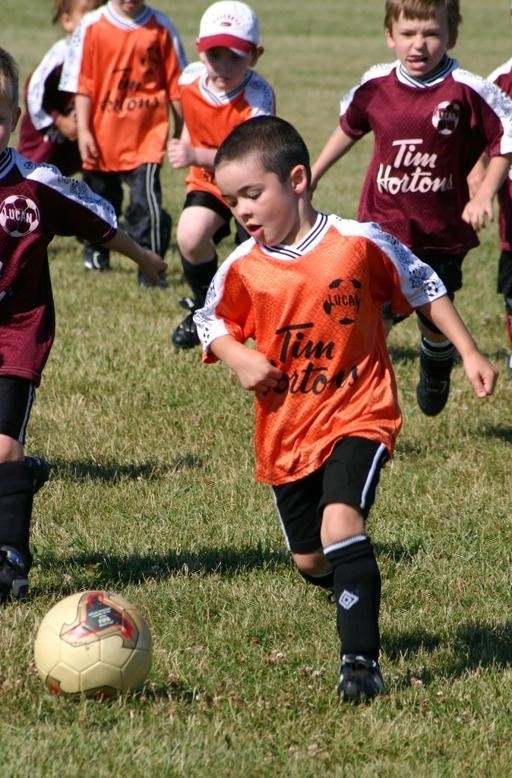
[138,269,167,289]
[25,454,50,495]
[0,550,29,601]
[82,248,113,273]
[336,651,384,704]
[417,352,454,414]
[171,298,205,347]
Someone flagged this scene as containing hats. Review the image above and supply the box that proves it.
[198,1,259,57]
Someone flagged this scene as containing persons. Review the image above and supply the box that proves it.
[166,0,275,349]
[466,55,512,375]
[18,0,109,177]
[191,113,499,706]
[60,0,190,290]
[308,0,512,417]
[0,47,169,606]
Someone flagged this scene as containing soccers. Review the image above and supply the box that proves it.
[34,589,153,702]
